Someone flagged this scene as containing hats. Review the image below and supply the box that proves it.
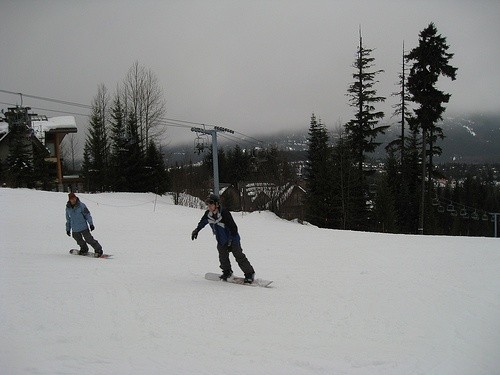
[68,193,76,199]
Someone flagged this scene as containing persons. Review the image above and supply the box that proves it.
[65,192,103,258]
[191,194,256,285]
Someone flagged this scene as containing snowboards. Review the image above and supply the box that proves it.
[204,272,274,288]
[69,248,114,259]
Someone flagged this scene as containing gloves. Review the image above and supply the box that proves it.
[192,228,199,240]
[67,231,71,236]
[90,225,94,231]
[225,241,233,251]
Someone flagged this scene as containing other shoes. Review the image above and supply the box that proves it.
[243,275,254,283]
[79,250,88,255]
[95,250,103,256]
[220,272,233,280]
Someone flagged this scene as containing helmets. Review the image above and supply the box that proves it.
[205,194,218,203]
